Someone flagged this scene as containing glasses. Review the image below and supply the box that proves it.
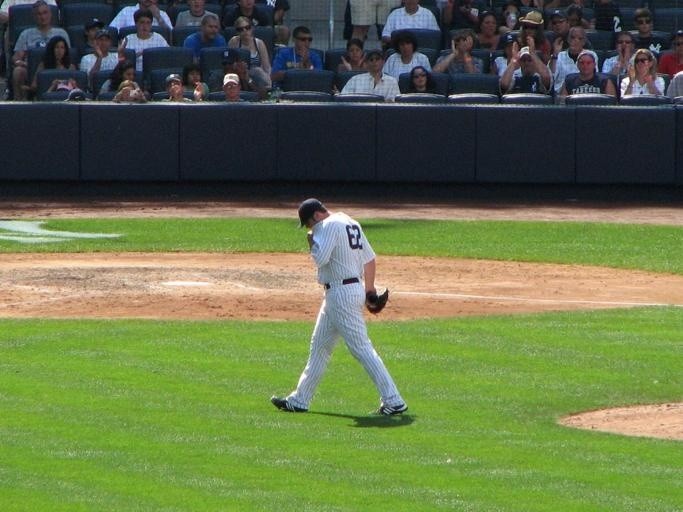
[525,25,537,30]
[296,37,313,42]
[552,18,565,25]
[617,40,632,44]
[414,72,428,78]
[235,24,251,32]
[638,19,653,25]
[633,58,650,63]
[580,60,593,65]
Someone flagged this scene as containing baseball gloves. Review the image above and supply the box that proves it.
[366,288,388,314]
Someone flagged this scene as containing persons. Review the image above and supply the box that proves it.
[1,0,323,104]
[270,199,407,418]
[330,1,682,98]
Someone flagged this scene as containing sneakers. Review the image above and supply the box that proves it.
[270,395,309,413]
[368,401,408,416]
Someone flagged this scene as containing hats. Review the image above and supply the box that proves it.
[297,198,322,229]
[501,33,519,47]
[86,17,106,28]
[550,9,569,19]
[221,48,240,65]
[520,45,531,59]
[95,29,112,40]
[518,11,544,26]
[365,49,386,60]
[165,73,183,85]
[672,27,683,36]
[223,72,240,87]
